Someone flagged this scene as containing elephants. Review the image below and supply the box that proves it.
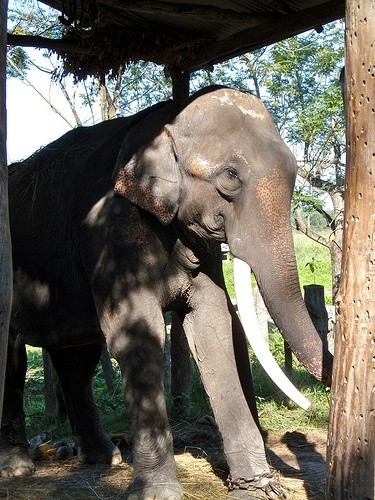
[6,83,334,500]
